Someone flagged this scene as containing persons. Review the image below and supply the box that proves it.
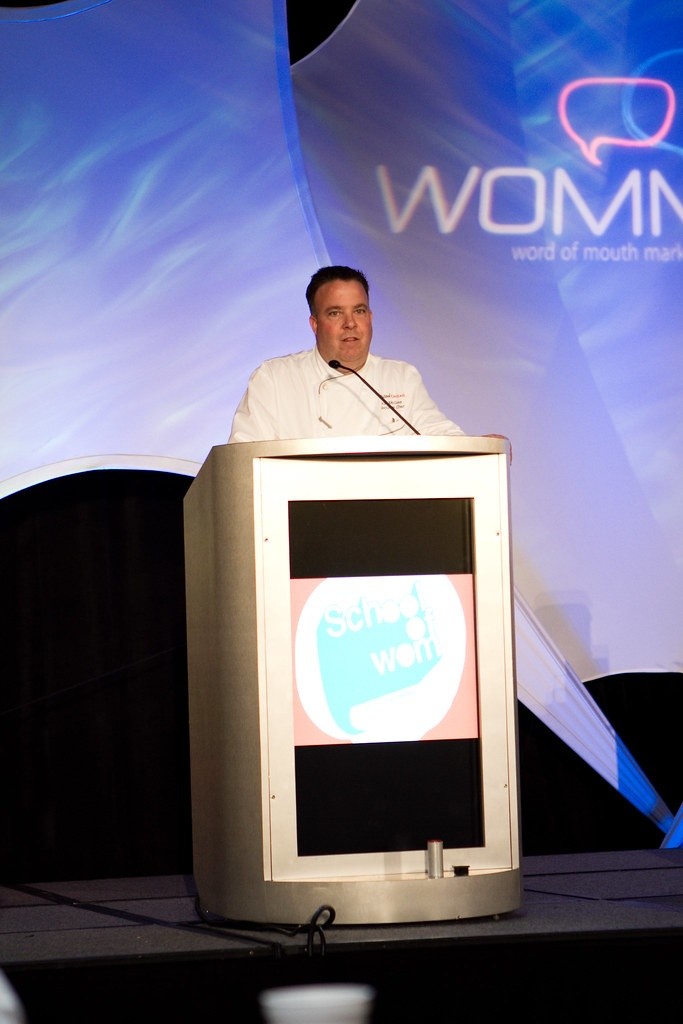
[228,266,466,444]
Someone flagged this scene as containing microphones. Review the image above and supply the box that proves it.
[328,360,418,436]
